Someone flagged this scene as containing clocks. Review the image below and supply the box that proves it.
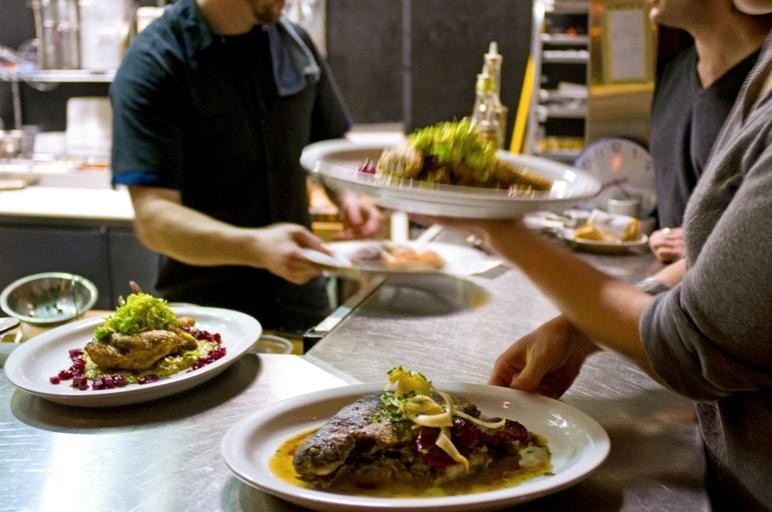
[573,135,657,219]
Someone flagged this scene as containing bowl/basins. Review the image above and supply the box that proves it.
[0,272,99,328]
[1,129,36,160]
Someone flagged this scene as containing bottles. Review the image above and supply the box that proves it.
[471,41,509,145]
[469,74,503,148]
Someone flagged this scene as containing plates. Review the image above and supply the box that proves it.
[557,227,649,253]
[299,131,604,220]
[2,306,263,407]
[302,238,494,277]
[221,380,612,512]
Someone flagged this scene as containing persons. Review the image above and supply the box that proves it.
[406,0,772,512]
[647,0,770,266]
[109,1,383,338]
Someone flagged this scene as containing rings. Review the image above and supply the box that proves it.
[661,227,671,235]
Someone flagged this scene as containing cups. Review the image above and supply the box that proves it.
[249,334,294,354]
[607,195,642,219]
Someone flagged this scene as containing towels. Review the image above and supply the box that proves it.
[267,21,323,98]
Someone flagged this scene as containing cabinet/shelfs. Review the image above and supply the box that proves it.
[522,0,593,167]
